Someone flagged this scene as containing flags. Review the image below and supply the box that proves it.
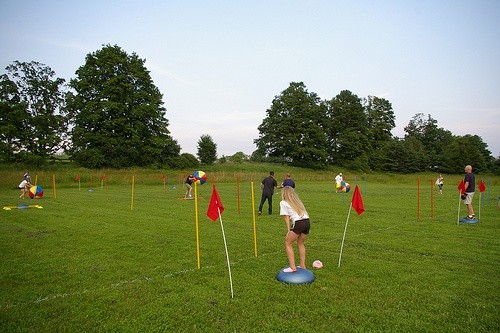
[207,184,224,221]
[478,179,486,192]
[458,179,466,194]
[351,185,366,215]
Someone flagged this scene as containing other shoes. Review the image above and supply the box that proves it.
[284,268,297,273]
[297,266,305,269]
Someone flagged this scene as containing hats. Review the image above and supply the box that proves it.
[277,179,295,189]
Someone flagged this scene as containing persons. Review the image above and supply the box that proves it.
[335,172,343,193]
[258,171,281,215]
[185,173,196,198]
[281,173,295,188]
[435,174,444,194]
[279,186,311,273]
[19,172,33,200]
[461,165,476,221]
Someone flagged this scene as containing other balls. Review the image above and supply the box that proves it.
[312,260,323,268]
[340,182,351,193]
[192,170,207,185]
[29,186,44,199]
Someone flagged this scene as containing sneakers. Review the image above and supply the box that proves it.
[472,214,476,220]
[464,216,473,221]
